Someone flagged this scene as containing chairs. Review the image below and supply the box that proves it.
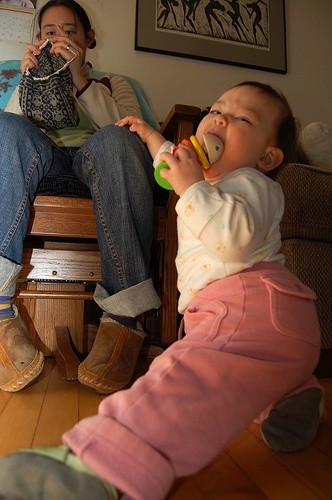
[0,59,201,351]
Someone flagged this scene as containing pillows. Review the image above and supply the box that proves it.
[298,120,332,171]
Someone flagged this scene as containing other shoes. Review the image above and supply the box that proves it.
[260,390,326,454]
[0,303,44,393]
[0,446,117,500]
[78,314,145,393]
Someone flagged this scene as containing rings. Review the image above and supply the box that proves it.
[66,44,70,50]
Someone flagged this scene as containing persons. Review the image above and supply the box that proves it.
[0,81,324,500]
[0,0,161,393]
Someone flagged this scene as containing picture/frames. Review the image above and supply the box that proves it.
[132,0,287,75]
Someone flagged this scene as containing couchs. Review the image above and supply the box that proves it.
[269,161,332,350]
